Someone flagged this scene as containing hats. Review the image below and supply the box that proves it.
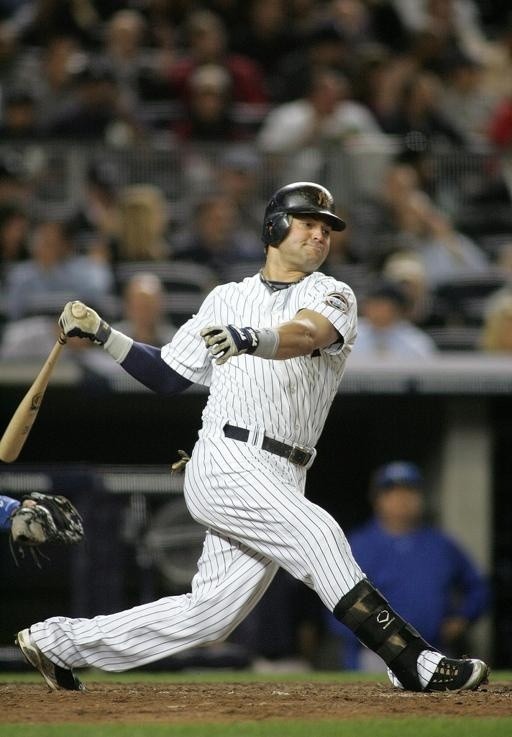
[366,459,429,498]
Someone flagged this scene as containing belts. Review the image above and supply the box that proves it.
[222,422,313,465]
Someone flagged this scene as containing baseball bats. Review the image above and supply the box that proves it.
[0,303,87,462]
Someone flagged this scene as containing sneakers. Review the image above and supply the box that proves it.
[390,645,488,692]
[15,628,85,692]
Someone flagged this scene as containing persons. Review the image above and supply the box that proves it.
[1,0,510,376]
[312,453,492,676]
[9,175,494,696]
[1,488,89,574]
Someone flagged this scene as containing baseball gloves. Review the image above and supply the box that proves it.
[10,492,85,591]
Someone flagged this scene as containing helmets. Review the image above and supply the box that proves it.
[262,181,346,251]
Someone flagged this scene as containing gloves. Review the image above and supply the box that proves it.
[57,301,132,364]
[200,324,280,367]
